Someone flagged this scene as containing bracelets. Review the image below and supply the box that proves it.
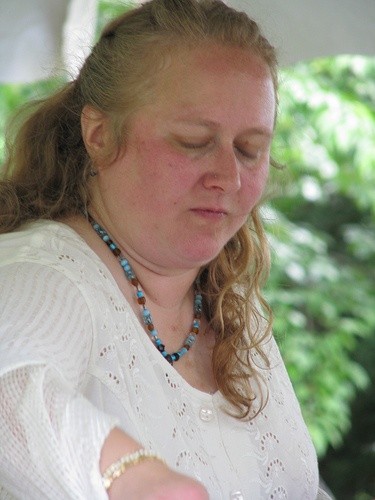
[100,449,166,489]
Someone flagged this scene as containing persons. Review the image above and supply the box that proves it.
[0,0,322,500]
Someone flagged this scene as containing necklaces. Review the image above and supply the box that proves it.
[78,206,204,363]
